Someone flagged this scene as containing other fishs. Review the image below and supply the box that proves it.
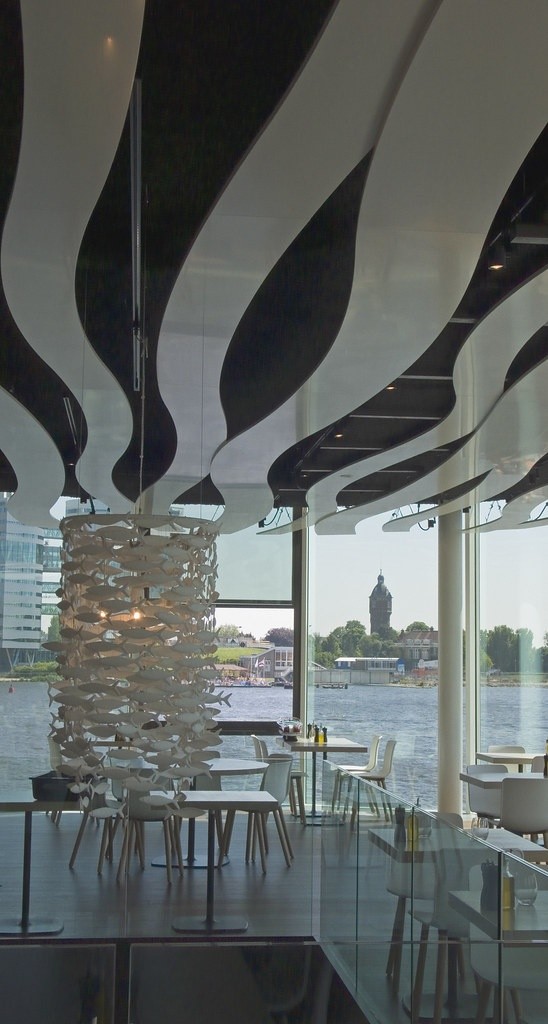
[39,520,234,823]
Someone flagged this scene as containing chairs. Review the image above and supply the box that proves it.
[68,734,548,1024]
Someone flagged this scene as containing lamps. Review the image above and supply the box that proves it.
[51,76,235,823]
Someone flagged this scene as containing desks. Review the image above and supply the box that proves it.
[448,890,548,940]
[368,828,548,1024]
[276,738,367,817]
[460,773,548,788]
[151,758,269,869]
[1,792,279,932]
[477,753,546,773]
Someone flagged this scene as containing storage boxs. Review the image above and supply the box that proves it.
[28,770,107,801]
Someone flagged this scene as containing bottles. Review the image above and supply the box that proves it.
[394,804,406,843]
[307,720,328,747]
[501,858,515,911]
[406,810,419,841]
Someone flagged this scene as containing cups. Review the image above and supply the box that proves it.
[515,871,539,906]
[470,817,489,840]
[479,862,499,912]
[419,815,432,839]
